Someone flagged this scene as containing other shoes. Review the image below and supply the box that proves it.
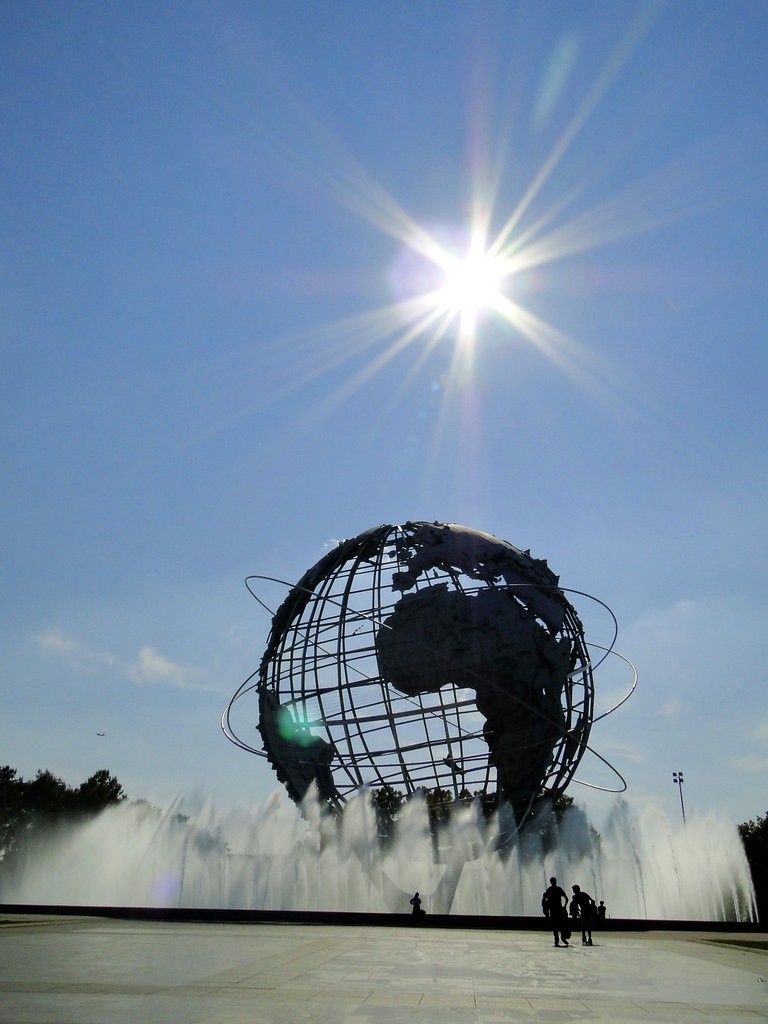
[587,938,592,944]
[562,938,569,944]
[582,938,587,945]
[555,944,559,947]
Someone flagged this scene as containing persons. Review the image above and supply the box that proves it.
[593,901,608,929]
[569,894,581,931]
[410,892,421,914]
[542,893,550,919]
[546,877,568,946]
[572,885,595,946]
